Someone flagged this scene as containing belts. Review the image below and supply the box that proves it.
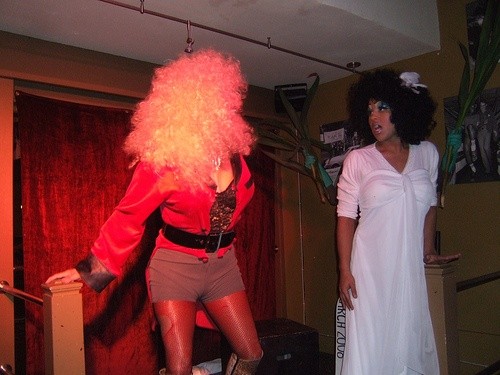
[161,224,235,252]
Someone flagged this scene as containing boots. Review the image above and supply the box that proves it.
[225,351,263,374]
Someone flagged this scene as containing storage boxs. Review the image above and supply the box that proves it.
[220,318,320,375]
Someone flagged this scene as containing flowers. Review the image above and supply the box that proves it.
[400,71,427,95]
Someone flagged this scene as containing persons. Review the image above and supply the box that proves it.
[334,69,462,375]
[46,48,265,375]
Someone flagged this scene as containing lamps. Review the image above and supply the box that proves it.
[184,20,194,54]
